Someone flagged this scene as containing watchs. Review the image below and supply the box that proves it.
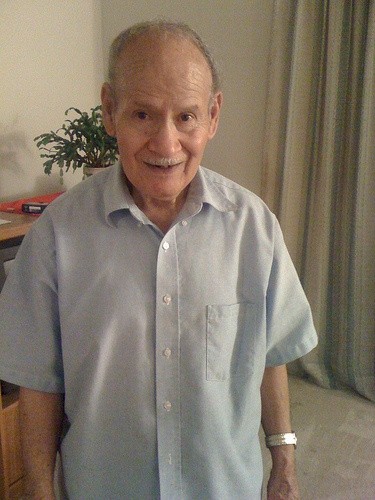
[265,429,298,452]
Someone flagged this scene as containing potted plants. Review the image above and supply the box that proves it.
[34,105,119,186]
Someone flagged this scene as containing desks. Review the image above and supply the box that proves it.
[1,191,66,240]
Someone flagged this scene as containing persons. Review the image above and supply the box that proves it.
[0,13,318,500]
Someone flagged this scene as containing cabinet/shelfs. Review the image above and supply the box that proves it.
[1,380,25,499]
